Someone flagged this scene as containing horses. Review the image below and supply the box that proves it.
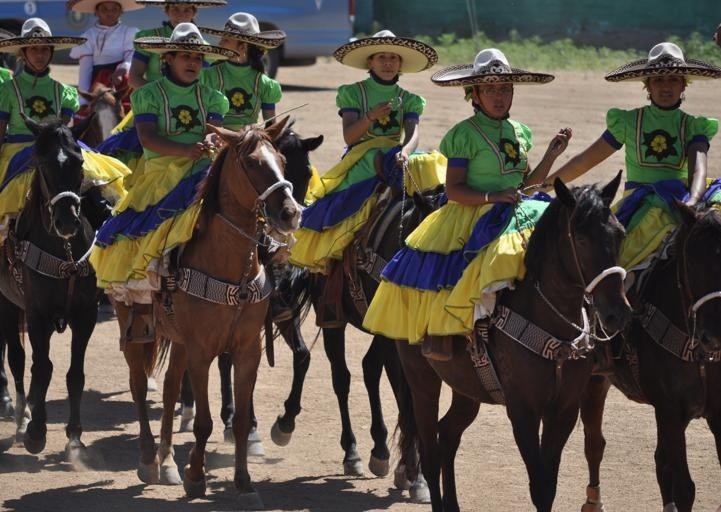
[103,113,291,506]
[270,184,450,504]
[580,194,721,512]
[0,112,102,472]
[392,169,634,512]
[179,117,324,447]
[69,81,133,314]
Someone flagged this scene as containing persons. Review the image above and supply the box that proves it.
[287,43,448,277]
[199,12,282,160]
[0,18,132,249]
[359,69,577,346]
[64,0,147,109]
[524,64,721,297]
[89,23,229,306]
[94,1,227,172]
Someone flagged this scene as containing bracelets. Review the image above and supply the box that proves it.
[366,111,374,123]
[387,95,402,112]
[484,191,491,202]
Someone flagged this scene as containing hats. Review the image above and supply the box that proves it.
[606,43,720,81]
[135,23,241,60]
[332,29,438,73]
[1,18,87,56]
[196,12,286,50]
[431,48,555,87]
[69,0,228,13]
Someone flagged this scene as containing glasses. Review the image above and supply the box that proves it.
[477,84,514,96]
